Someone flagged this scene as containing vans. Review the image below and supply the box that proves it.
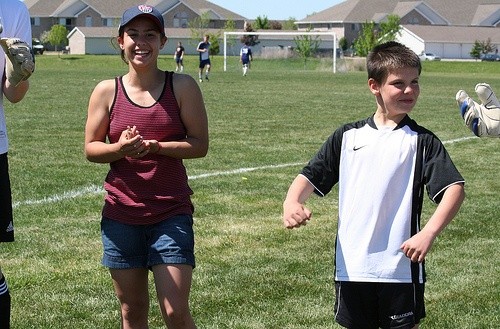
[32,38,45,54]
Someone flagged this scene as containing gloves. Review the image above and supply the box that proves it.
[1,38,36,88]
[454,82,500,136]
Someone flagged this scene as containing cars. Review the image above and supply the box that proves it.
[335,47,360,57]
[478,52,500,60]
[417,52,442,62]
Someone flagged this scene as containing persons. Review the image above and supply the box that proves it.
[85,8,210,329]
[173,41,185,73]
[282,42,466,329]
[239,42,254,76]
[196,36,214,83]
[0,0,36,329]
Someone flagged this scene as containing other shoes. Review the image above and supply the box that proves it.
[199,78,203,83]
[205,77,209,82]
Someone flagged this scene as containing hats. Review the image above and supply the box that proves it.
[117,4,166,38]
